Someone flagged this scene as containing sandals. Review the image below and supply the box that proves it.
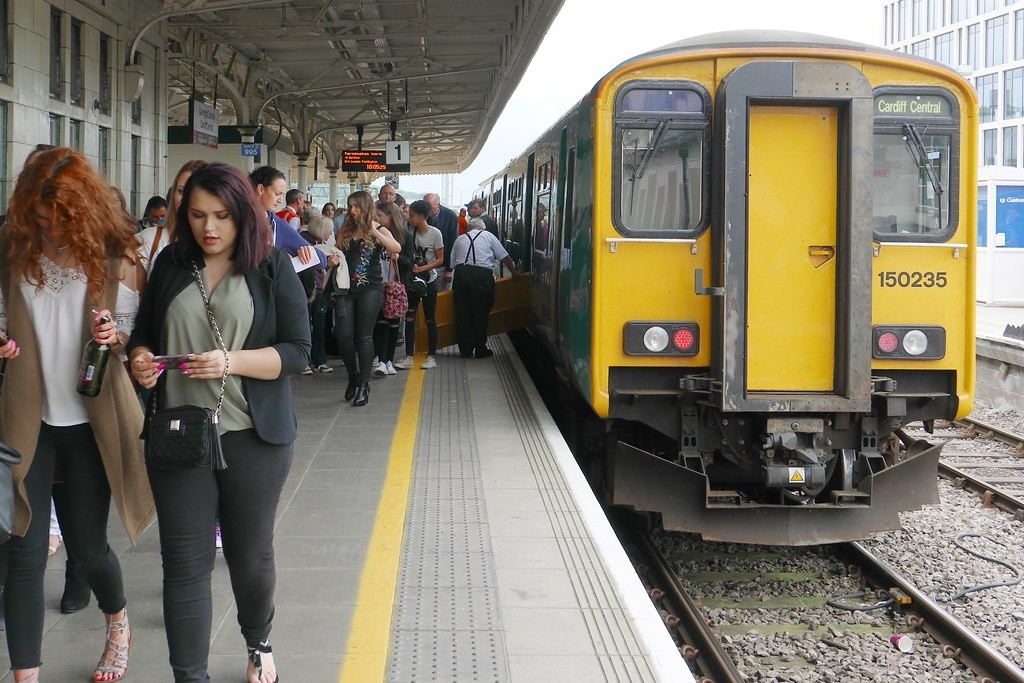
[93,609,131,683]
[246,637,279,683]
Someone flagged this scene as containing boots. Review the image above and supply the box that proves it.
[342,359,370,407]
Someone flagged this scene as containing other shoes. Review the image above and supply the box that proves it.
[393,356,414,369]
[299,366,313,374]
[60,560,91,613]
[314,363,334,373]
[372,355,379,367]
[374,360,396,375]
[420,356,437,368]
[463,349,492,358]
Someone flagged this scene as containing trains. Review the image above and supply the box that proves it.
[473,28,979,544]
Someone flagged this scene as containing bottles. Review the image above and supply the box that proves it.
[77,317,110,397]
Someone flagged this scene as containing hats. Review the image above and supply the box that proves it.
[464,200,472,208]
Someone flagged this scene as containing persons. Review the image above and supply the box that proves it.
[126,161,312,683]
[507,201,547,270]
[45,159,523,614]
[0,146,156,683]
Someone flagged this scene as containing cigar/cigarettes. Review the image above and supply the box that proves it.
[92,309,109,321]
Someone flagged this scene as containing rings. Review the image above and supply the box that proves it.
[0,355,3,359]
[138,377,143,385]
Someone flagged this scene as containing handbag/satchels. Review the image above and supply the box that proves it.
[383,252,408,319]
[0,441,23,545]
[146,405,212,469]
[403,255,431,297]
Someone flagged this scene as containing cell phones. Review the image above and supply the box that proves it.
[151,354,193,370]
